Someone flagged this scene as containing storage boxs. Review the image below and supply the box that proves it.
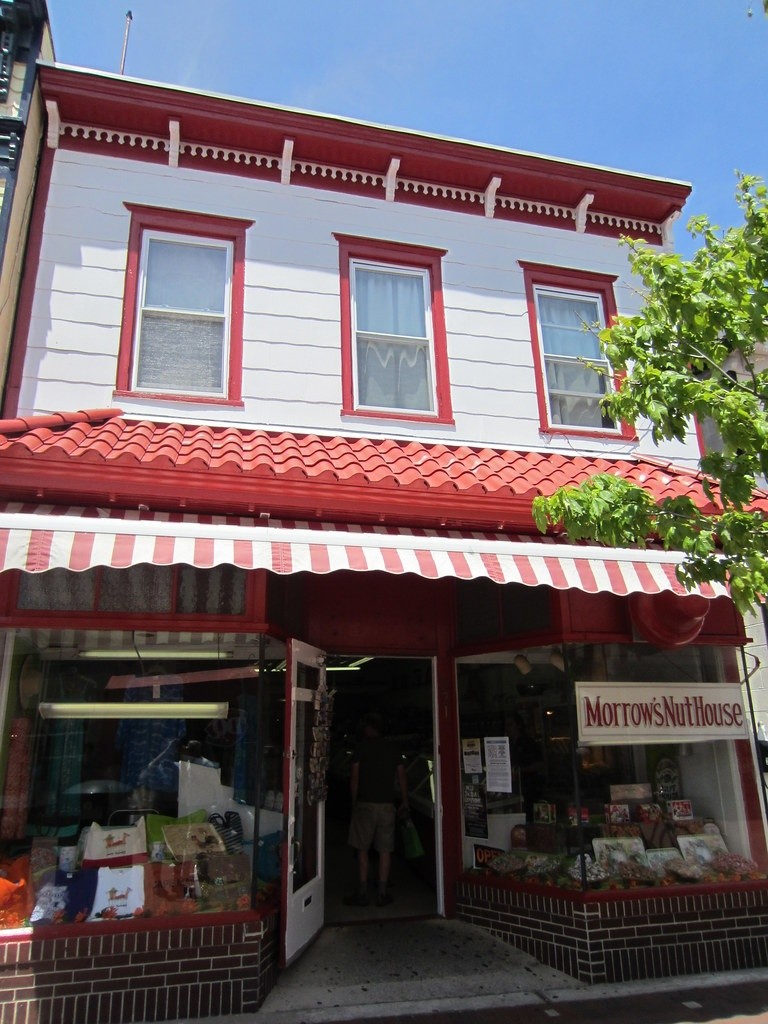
[533,799,692,826]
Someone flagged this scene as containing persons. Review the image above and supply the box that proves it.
[505,712,545,822]
[344,714,408,907]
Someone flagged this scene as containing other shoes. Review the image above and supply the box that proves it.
[376,892,393,907]
[343,891,368,906]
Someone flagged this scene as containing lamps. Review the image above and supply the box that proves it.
[77,647,235,660]
[39,635,229,718]
[549,650,577,672]
[513,654,532,675]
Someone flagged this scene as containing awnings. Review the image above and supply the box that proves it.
[2,499,768,603]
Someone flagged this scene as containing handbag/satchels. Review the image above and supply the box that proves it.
[400,819,425,858]
[208,810,242,854]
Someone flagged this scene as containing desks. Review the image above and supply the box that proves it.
[524,821,714,854]
